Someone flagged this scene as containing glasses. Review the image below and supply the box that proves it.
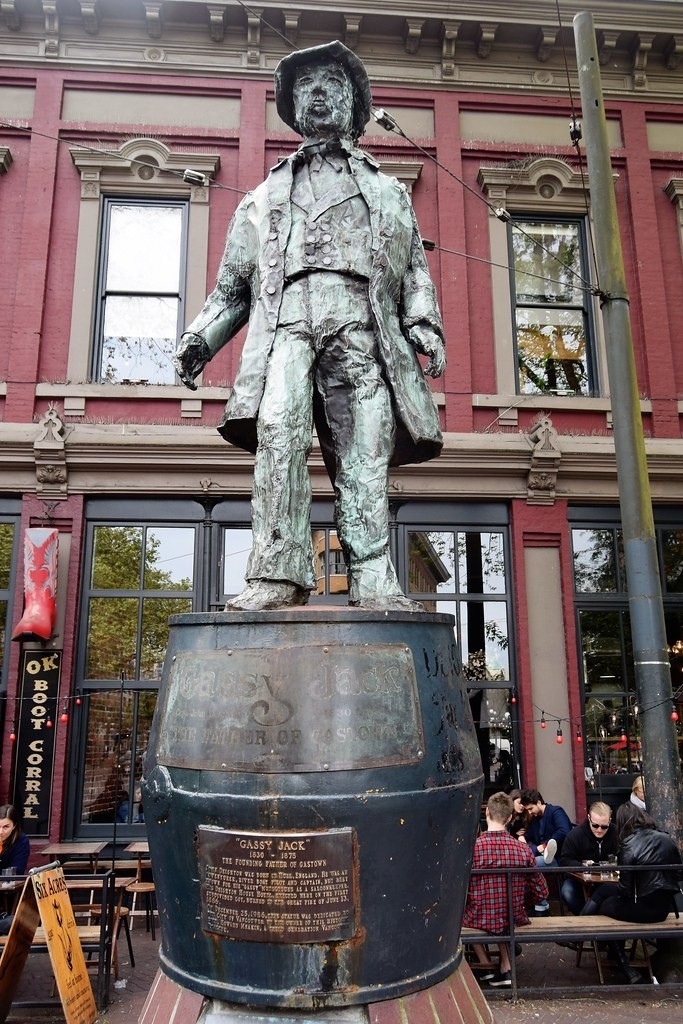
[590,816,610,830]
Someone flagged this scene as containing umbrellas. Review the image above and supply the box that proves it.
[604,737,640,752]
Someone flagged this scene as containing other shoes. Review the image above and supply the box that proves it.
[535,902,549,911]
[543,839,558,864]
[489,970,511,986]
[478,970,495,981]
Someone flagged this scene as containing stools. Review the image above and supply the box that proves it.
[0,882,157,1007]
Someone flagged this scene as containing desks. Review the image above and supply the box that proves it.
[2,876,139,998]
[33,842,109,925]
[123,841,150,929]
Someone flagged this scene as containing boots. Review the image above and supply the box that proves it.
[607,940,641,984]
[555,897,600,950]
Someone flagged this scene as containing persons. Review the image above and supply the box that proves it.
[172,39,448,608]
[457,776,683,988]
[599,763,624,774]
[0,805,30,916]
[88,774,131,823]
